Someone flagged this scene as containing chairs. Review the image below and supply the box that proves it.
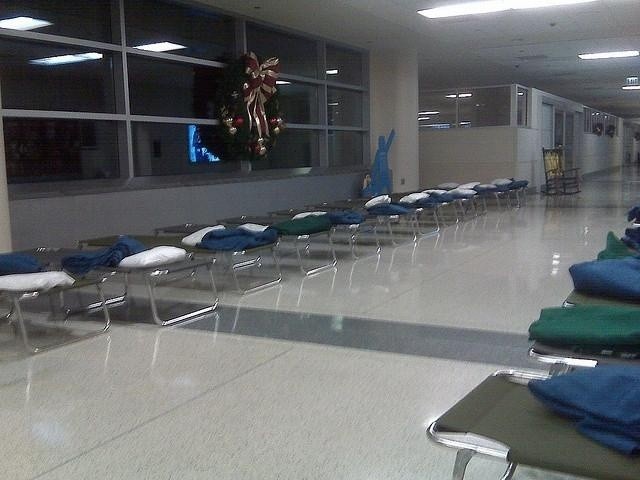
[541,145,581,195]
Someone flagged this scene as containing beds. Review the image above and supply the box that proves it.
[218,214,380,258]
[267,211,417,247]
[76,234,283,293]
[159,224,338,275]
[427,370,638,478]
[20,248,222,325]
[526,207,640,377]
[305,180,527,235]
[2,252,116,353]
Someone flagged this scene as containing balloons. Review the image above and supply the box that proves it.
[222,113,282,156]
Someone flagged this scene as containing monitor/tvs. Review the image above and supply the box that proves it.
[186,124,224,164]
